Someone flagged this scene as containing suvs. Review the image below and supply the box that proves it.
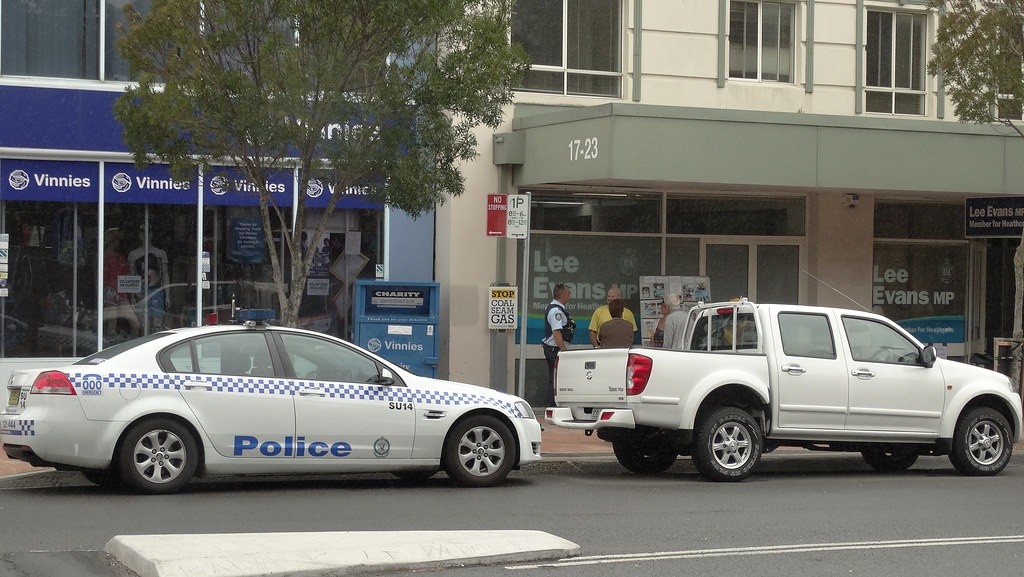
[89,278,290,351]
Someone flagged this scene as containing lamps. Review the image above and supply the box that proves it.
[575,200,604,218]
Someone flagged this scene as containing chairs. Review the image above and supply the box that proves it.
[221,343,252,376]
[251,348,274,378]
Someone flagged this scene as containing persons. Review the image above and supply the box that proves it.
[598,303,634,349]
[302,232,330,265]
[104,227,140,339]
[127,223,170,336]
[651,293,694,349]
[587,288,638,348]
[542,284,576,407]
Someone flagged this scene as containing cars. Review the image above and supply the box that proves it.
[0,318,544,494]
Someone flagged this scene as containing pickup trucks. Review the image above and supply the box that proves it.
[546,296,1022,482]
